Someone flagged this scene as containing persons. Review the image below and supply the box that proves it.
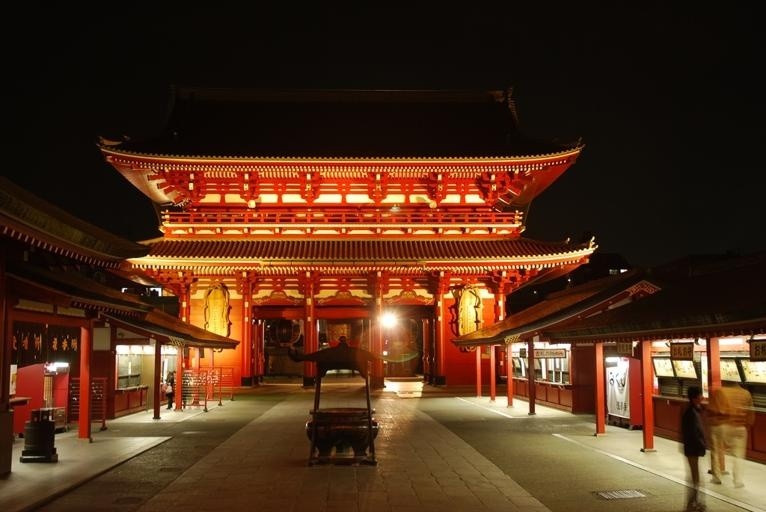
[709,381,755,488]
[681,386,707,511]
[165,369,188,410]
